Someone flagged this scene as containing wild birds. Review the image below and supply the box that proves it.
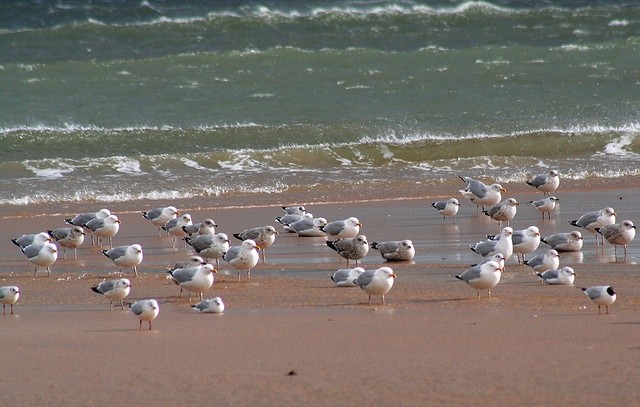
[141,207,180,237]
[0,286,20,314]
[482,198,520,227]
[317,217,362,239]
[189,297,224,313]
[181,219,218,238]
[91,279,132,312]
[470,253,505,270]
[284,217,327,237]
[576,285,618,315]
[166,256,207,298]
[184,233,231,269]
[129,299,159,331]
[455,261,502,300]
[469,227,513,262]
[46,226,85,260]
[526,169,560,197]
[457,175,506,212]
[595,220,636,254]
[166,264,217,303]
[274,213,313,233]
[63,209,111,245]
[540,230,584,251]
[352,267,397,303]
[431,198,460,218]
[369,240,416,261]
[530,196,560,220]
[325,234,369,268]
[11,232,52,268]
[100,243,143,278]
[233,226,279,262]
[161,214,193,248]
[486,226,540,265]
[568,207,617,244]
[524,249,561,274]
[330,267,366,287]
[20,244,58,277]
[281,206,306,216]
[537,266,577,285]
[219,239,260,282]
[82,215,121,249]
